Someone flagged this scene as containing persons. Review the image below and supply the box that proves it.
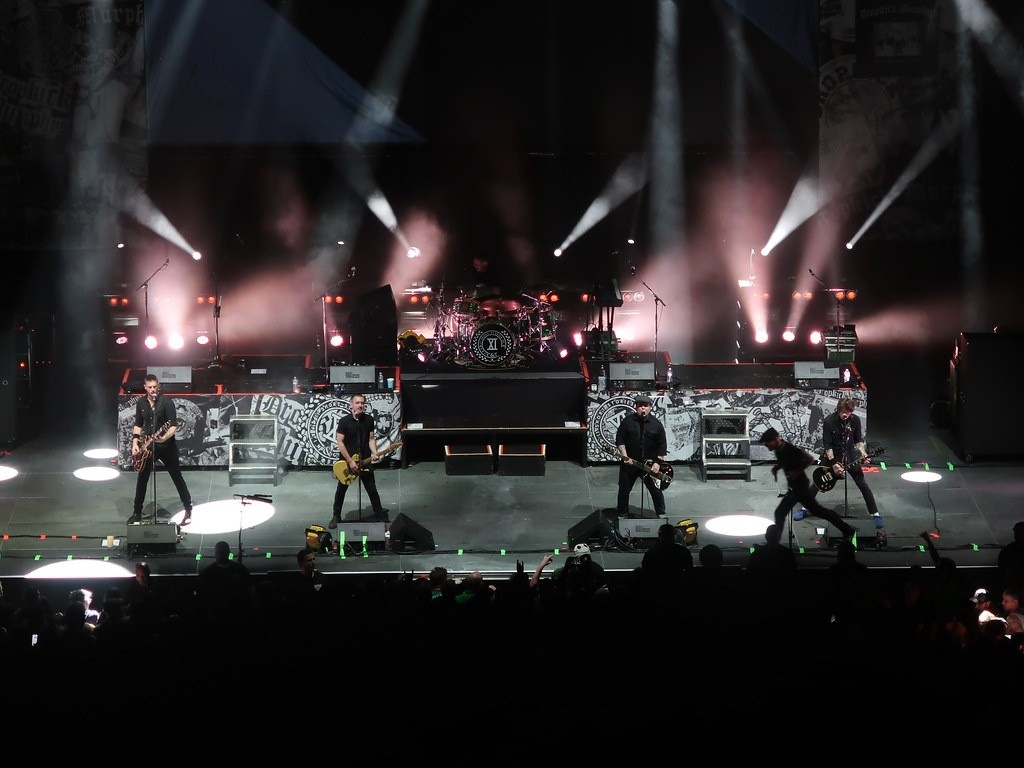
[616,396,667,519]
[759,428,856,542]
[126,374,193,525]
[473,255,503,304]
[1,521,1024,768]
[329,394,390,529]
[794,399,883,528]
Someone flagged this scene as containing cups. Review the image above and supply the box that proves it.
[387,378,394,389]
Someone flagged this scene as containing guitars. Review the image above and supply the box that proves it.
[812,447,885,492]
[130,418,173,472]
[602,446,674,491]
[332,441,405,486]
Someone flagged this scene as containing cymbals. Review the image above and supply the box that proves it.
[481,299,521,312]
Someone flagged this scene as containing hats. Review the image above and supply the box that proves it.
[759,427,778,442]
[969,588,991,603]
[634,395,654,405]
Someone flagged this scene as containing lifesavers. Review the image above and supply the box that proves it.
[489,305,527,343]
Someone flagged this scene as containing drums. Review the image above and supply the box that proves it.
[522,303,555,341]
[452,297,481,317]
[470,318,514,366]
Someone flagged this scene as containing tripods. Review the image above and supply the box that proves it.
[517,307,564,368]
[422,292,477,367]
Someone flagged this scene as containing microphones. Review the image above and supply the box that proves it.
[355,412,359,421]
[843,413,847,420]
[152,394,157,402]
[641,410,646,418]
[234,494,273,504]
[630,265,635,275]
[351,267,356,277]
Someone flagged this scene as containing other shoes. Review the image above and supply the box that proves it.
[329,517,341,529]
[844,526,855,543]
[126,514,141,525]
[182,507,193,525]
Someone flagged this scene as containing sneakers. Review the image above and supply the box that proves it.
[794,509,807,520]
[873,513,883,529]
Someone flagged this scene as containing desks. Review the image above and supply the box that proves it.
[117,390,404,470]
[588,386,867,468]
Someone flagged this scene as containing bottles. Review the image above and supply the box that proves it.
[378,372,384,389]
[667,367,673,382]
[590,373,606,393]
[599,365,605,377]
[844,369,850,383]
[293,377,298,393]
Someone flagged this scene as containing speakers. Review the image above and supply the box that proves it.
[608,363,655,390]
[568,509,619,550]
[390,512,435,555]
[336,522,386,552]
[330,365,375,395]
[826,517,878,546]
[793,360,839,391]
[592,279,623,308]
[147,366,192,394]
[615,518,668,549]
[127,522,177,557]
